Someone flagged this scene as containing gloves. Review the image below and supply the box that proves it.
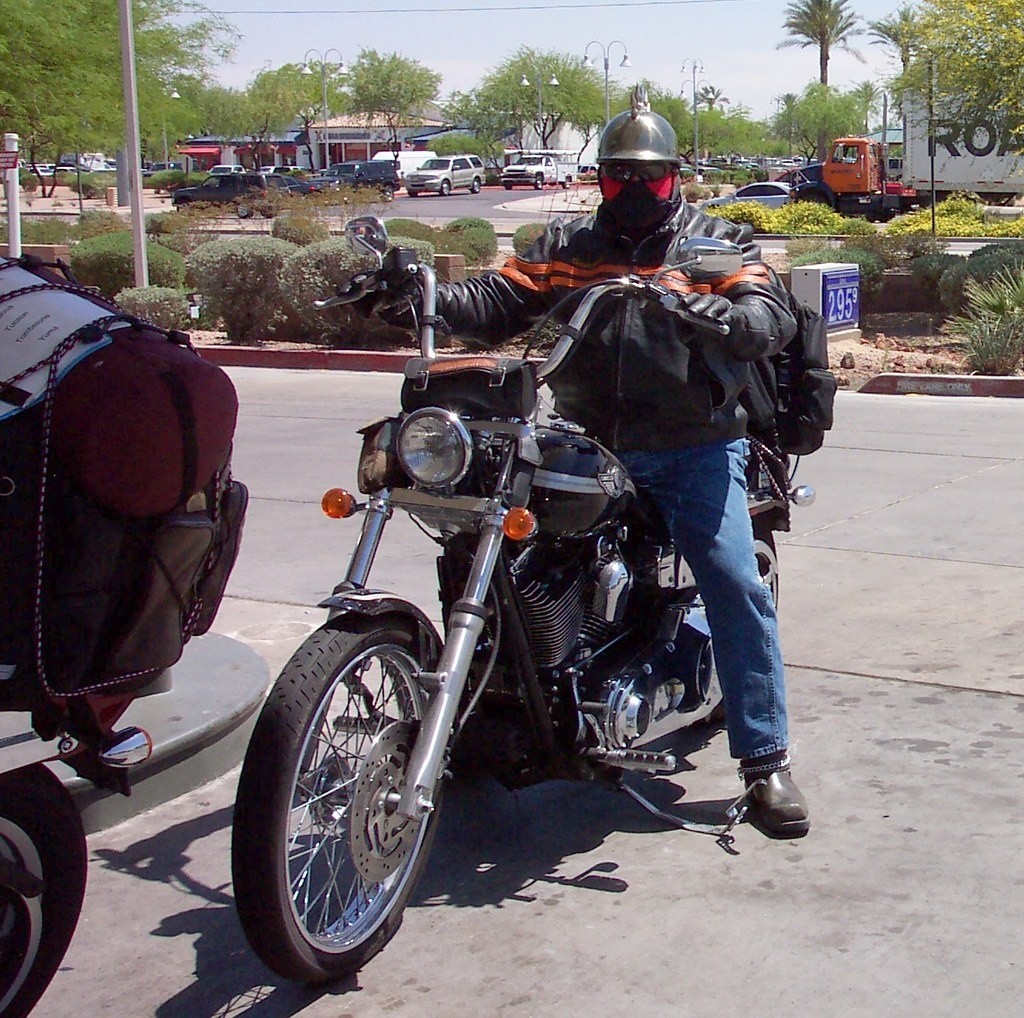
[339,270,403,314]
[680,291,745,338]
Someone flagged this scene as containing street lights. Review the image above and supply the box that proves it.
[581,40,632,125]
[520,63,560,150]
[162,87,181,170]
[301,47,349,173]
[678,57,716,183]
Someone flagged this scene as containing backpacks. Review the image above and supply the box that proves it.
[768,288,838,454]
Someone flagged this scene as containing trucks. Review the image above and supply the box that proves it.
[818,73,1023,223]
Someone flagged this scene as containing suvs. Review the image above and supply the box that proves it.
[405,154,486,197]
[170,172,292,218]
[299,159,401,201]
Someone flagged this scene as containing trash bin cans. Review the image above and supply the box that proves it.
[106,186,118,208]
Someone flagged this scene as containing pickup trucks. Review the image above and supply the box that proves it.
[498,150,580,191]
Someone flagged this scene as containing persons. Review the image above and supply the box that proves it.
[338,85,813,838]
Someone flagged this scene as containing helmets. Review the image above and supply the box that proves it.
[595,85,681,167]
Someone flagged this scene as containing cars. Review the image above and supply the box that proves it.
[17,152,183,177]
[681,155,858,183]
[206,164,327,192]
[580,163,601,174]
[700,180,789,211]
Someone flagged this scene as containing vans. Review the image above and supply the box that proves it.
[371,151,439,180]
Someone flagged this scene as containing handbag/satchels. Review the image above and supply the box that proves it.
[1,254,248,696]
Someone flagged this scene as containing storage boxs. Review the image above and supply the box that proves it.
[793,261,860,333]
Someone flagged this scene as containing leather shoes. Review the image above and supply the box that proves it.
[739,757,811,833]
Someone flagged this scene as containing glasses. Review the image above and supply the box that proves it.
[598,162,677,183]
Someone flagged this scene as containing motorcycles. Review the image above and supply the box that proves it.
[1,478,245,1018]
[229,216,817,989]
